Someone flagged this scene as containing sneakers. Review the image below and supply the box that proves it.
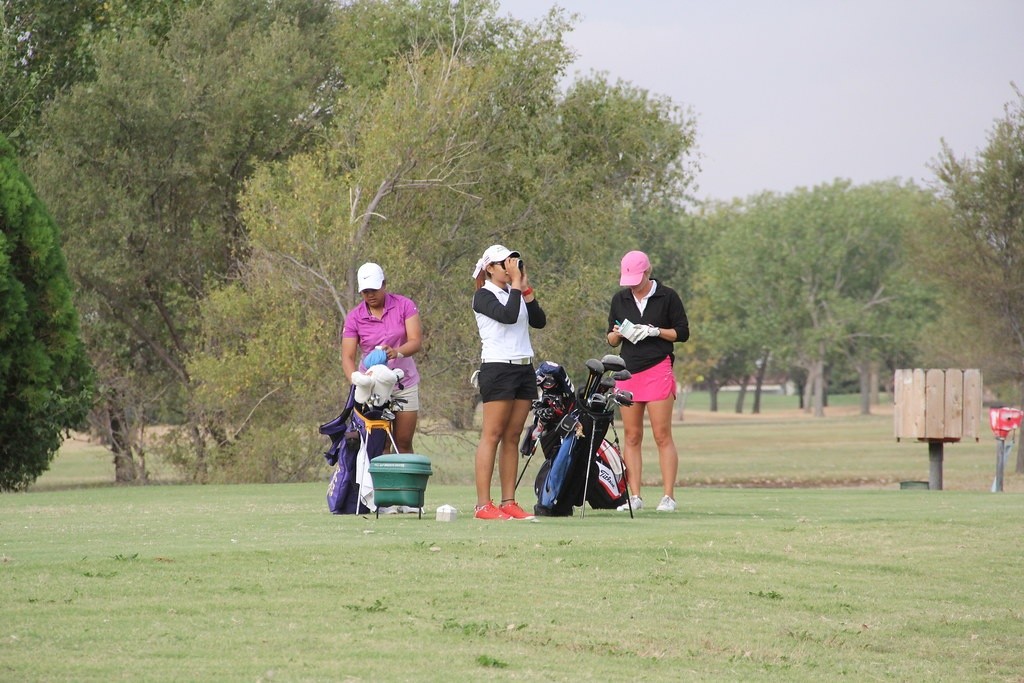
[473,499,513,520]
[616,494,643,512]
[499,503,535,521]
[655,495,678,513]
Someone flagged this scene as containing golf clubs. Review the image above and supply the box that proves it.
[531,369,566,423]
[366,394,409,420]
[581,352,635,414]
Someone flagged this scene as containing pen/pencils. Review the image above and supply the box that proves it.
[615,319,622,327]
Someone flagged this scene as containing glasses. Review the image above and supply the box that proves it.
[494,260,507,270]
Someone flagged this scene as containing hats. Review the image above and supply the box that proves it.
[620,250,650,286]
[481,244,521,271]
[358,262,385,294]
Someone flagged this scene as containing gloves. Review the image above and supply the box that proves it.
[633,324,660,340]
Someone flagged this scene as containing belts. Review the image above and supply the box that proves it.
[481,356,533,365]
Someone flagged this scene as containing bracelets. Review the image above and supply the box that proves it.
[522,286,532,297]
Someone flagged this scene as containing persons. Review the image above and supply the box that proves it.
[472,244,546,521]
[342,263,421,454]
[607,250,690,511]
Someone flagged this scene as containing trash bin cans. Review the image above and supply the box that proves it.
[894,368,982,443]
